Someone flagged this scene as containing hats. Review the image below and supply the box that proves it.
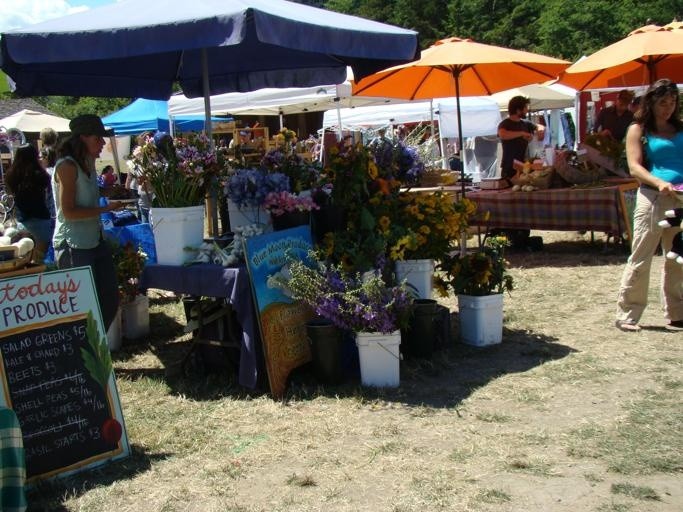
[69,114,111,139]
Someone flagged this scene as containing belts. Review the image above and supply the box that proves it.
[640,183,658,191]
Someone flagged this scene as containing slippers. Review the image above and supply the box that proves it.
[615,319,642,333]
[664,319,683,331]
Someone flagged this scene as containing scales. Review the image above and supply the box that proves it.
[480,178,512,189]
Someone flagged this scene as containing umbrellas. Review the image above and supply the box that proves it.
[0,0,422,200]
[345,33,572,177]
[551,18,683,104]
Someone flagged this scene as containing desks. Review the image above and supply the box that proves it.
[454,184,636,251]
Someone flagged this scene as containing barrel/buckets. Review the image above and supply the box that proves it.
[120,295,151,339]
[354,329,402,389]
[394,257,436,300]
[456,292,504,347]
[225,196,275,235]
[148,205,206,266]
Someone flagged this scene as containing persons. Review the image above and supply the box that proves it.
[590,90,634,146]
[613,78,682,334]
[99,163,117,193]
[367,128,391,154]
[49,114,127,343]
[131,131,152,221]
[2,139,54,264]
[495,95,547,241]
[239,129,253,145]
[38,127,56,159]
[472,135,499,175]
[123,138,141,223]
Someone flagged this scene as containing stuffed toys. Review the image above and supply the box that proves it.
[657,207,683,265]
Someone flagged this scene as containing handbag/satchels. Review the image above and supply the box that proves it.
[623,150,650,174]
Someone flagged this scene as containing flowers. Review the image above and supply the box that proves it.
[117,119,512,389]
